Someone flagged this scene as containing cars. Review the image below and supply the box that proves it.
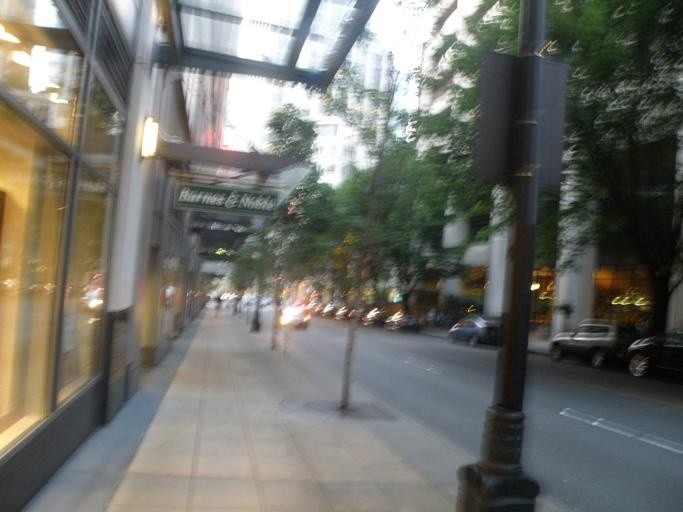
[274,305,313,331]
[295,298,424,336]
[619,325,681,381]
[445,314,502,348]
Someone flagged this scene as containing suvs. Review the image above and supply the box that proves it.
[548,314,632,373]
